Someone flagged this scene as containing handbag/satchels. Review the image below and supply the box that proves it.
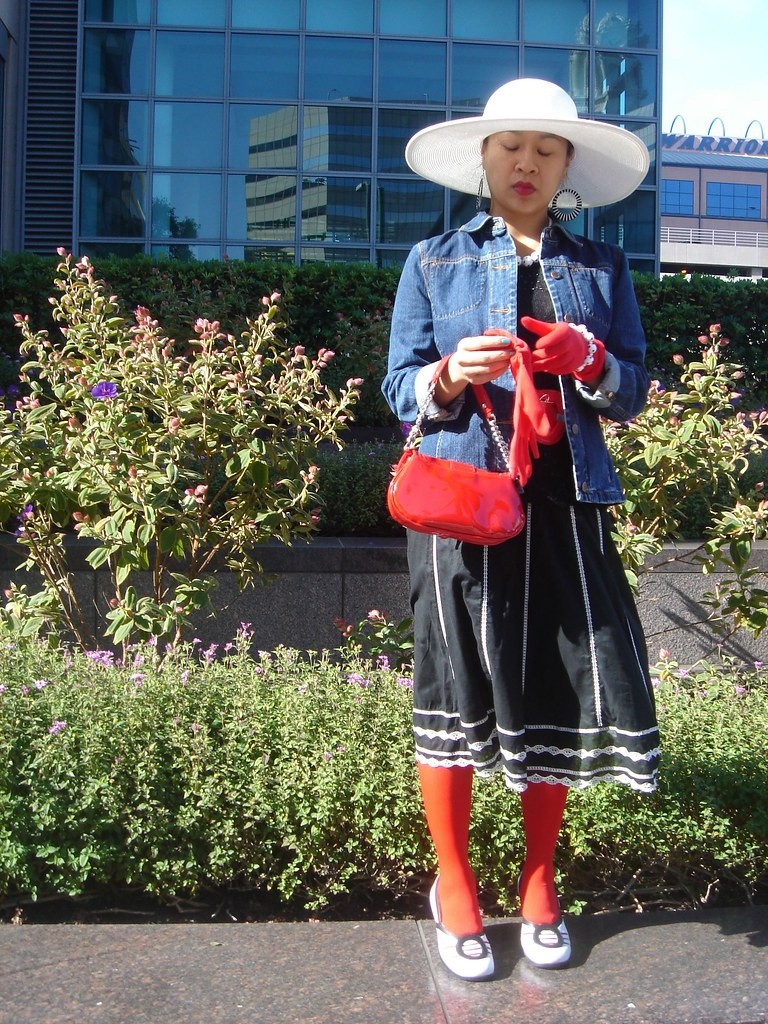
[387,354,525,546]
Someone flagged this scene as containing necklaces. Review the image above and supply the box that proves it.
[516,248,540,266]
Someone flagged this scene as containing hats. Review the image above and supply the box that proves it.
[404,78,650,209]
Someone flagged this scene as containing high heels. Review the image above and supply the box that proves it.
[429,875,495,982]
[517,870,572,969]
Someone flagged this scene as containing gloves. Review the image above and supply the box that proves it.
[521,317,606,382]
[482,329,550,486]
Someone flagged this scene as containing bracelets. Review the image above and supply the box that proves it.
[568,322,596,372]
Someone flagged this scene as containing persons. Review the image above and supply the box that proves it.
[379,78,662,979]
